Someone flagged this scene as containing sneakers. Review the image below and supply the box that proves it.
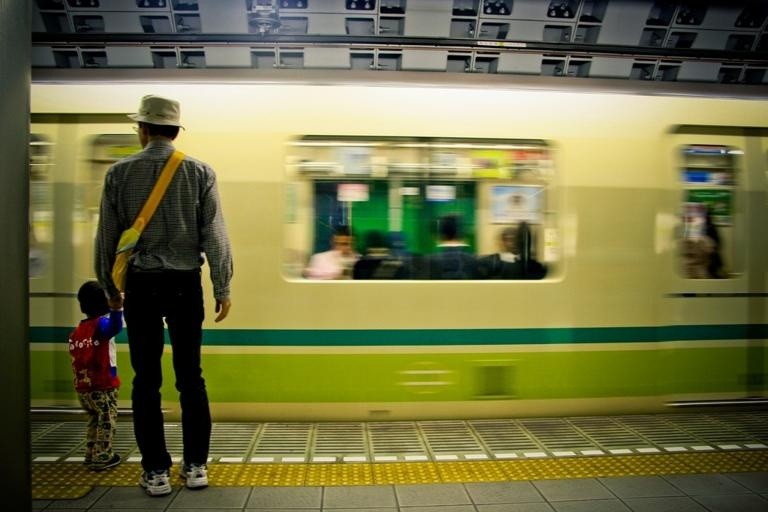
[179,461,208,489]
[83,453,122,471]
[138,469,172,495]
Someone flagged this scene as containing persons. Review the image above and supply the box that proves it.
[673,207,727,279]
[68,281,125,470]
[94,95,234,497]
[303,216,546,280]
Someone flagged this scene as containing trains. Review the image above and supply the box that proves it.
[28,67,768,422]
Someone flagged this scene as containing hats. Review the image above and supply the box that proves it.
[126,95,185,131]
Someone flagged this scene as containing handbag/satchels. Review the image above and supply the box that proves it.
[111,228,140,292]
